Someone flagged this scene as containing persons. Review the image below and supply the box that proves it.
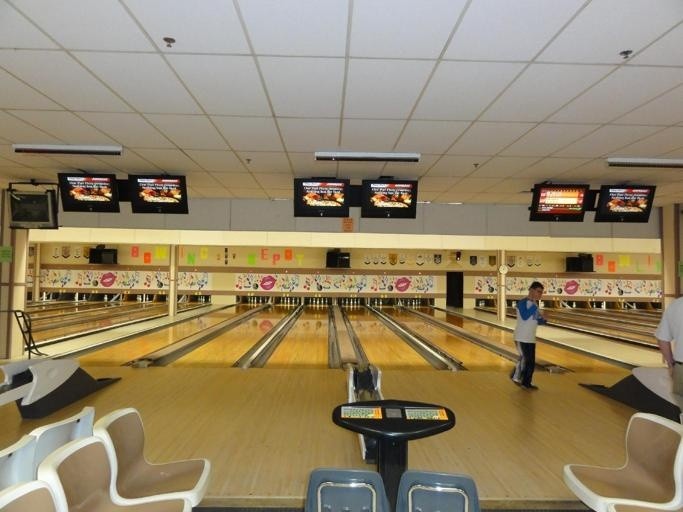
[655,296,682,397]
[510,282,547,391]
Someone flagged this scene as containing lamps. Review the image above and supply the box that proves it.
[10,141,122,158]
[314,151,421,164]
[607,157,683,169]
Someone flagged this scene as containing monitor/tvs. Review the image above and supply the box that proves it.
[127,174,188,213]
[293,178,350,217]
[362,179,417,218]
[593,184,657,223]
[9,191,57,229]
[529,183,589,222]
[57,172,116,210]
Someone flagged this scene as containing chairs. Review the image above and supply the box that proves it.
[607,503,674,512]
[561,412,682,512]
[0,404,211,511]
[390,472,479,512]
[302,468,394,512]
